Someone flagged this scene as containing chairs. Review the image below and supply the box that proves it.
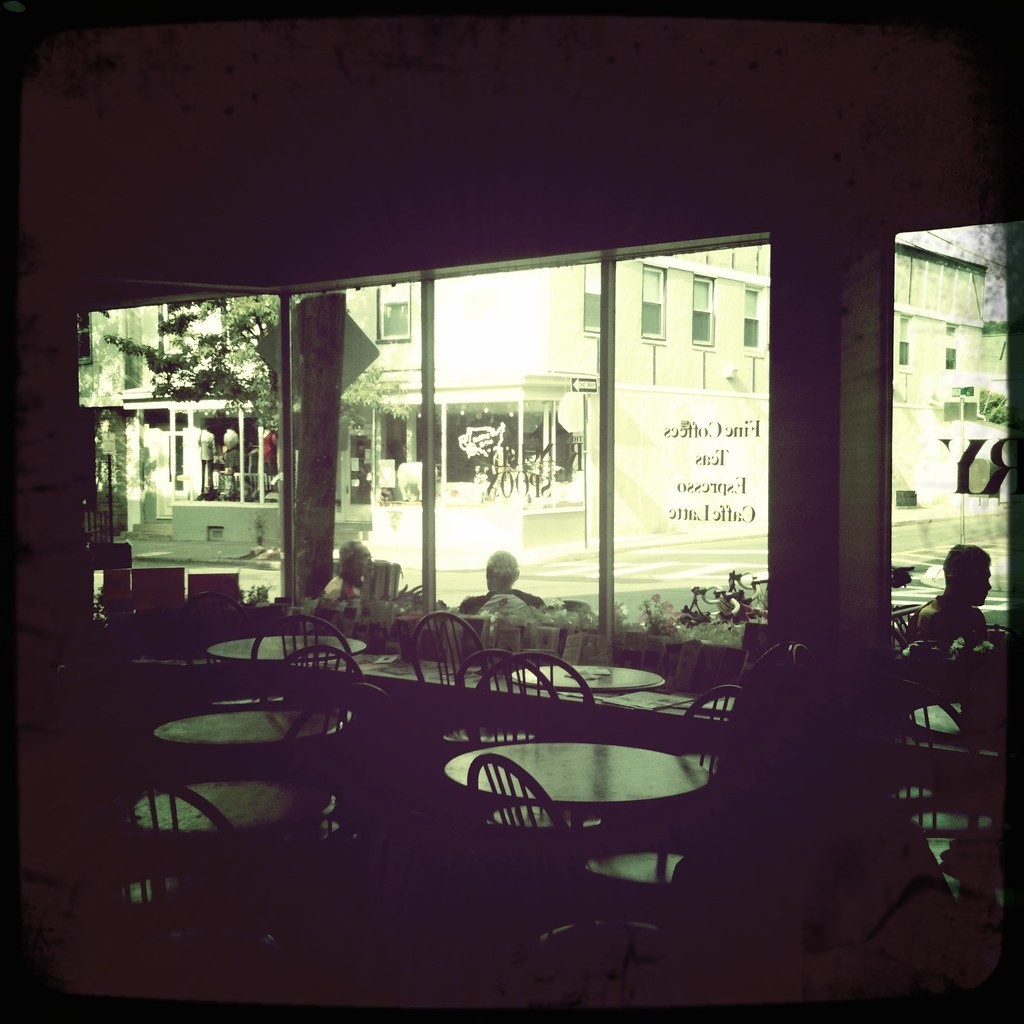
[110,591,1011,1007]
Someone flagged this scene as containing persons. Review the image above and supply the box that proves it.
[455,551,546,614]
[320,542,375,602]
[902,545,993,655]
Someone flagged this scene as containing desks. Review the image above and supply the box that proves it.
[202,633,368,695]
[506,652,667,734]
[127,779,338,870]
[439,742,702,849]
[152,708,355,779]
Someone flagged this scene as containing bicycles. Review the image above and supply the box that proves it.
[891,564,920,643]
[677,568,770,627]
